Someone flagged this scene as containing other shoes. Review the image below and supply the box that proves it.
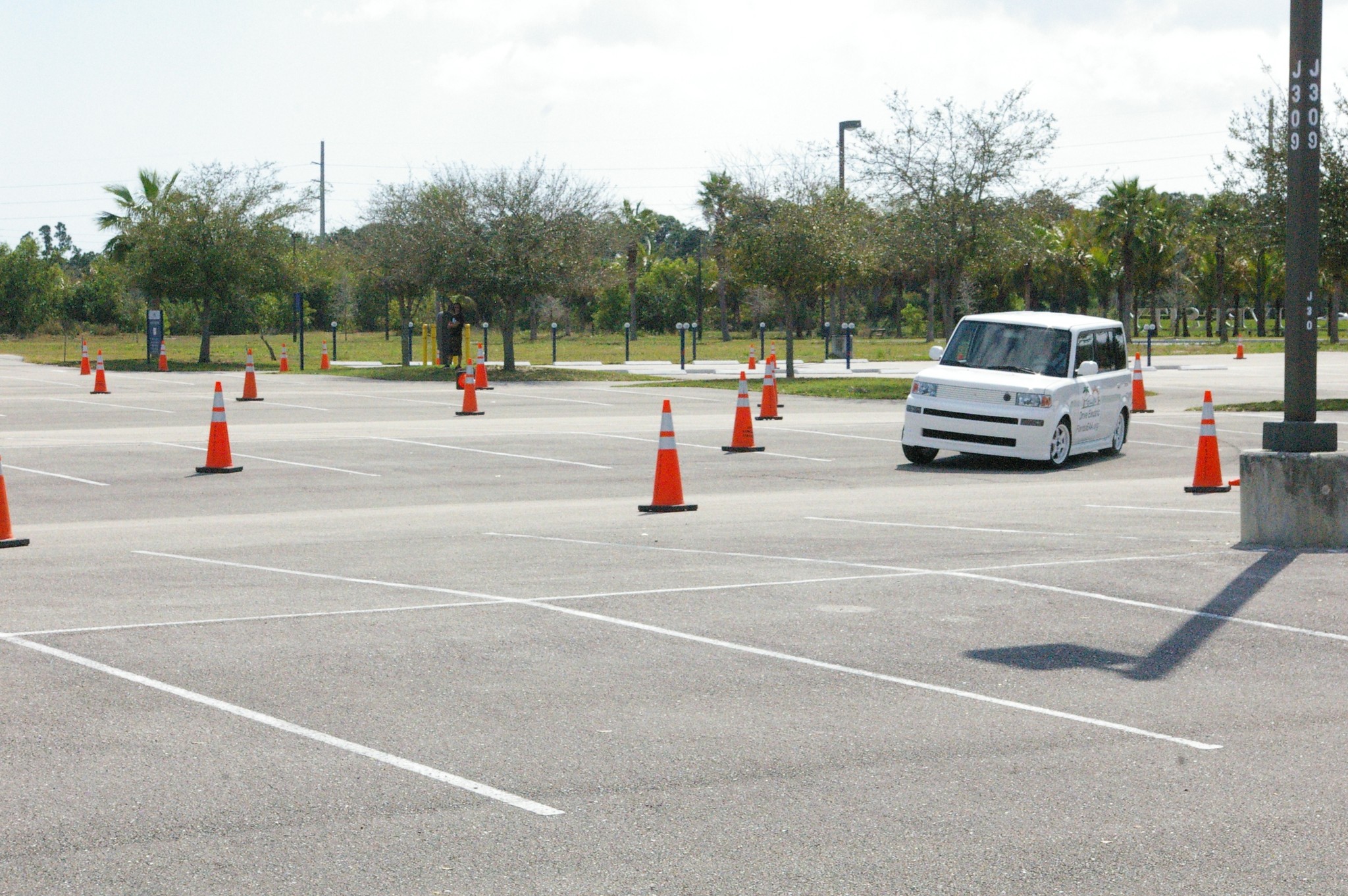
[443,364,450,369]
[454,365,461,370]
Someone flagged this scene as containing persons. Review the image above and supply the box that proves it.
[442,303,464,370]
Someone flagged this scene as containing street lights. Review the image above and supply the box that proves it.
[838,119,861,327]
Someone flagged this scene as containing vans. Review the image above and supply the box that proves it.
[900,310,1132,469]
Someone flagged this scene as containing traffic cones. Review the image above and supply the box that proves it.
[1184,390,1231,493]
[748,344,756,370]
[278,343,290,372]
[79,340,92,375]
[755,340,784,420]
[155,339,171,372]
[722,372,765,452]
[1129,352,1154,413]
[456,359,485,415]
[1229,479,1241,485]
[1234,334,1247,359]
[237,348,265,401]
[92,349,112,394]
[474,343,493,390]
[435,348,440,365]
[638,399,698,512]
[195,382,244,474]
[0,454,29,548]
[323,341,330,370]
